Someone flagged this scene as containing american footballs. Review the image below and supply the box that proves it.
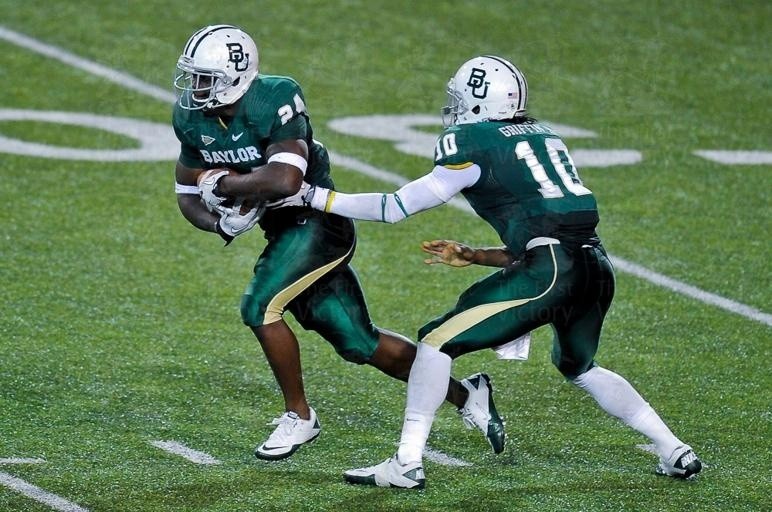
[198,170,254,214]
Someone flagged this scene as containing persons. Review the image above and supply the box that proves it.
[262,55,703,489]
[172,23,506,461]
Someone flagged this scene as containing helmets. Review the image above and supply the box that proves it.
[173,23,258,112]
[441,54,526,123]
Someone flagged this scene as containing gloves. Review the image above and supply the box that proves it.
[216,202,265,239]
[200,170,227,212]
[268,181,317,212]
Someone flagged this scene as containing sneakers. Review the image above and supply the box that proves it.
[460,371,506,454]
[343,455,423,488]
[256,408,322,462]
[655,447,701,478]
